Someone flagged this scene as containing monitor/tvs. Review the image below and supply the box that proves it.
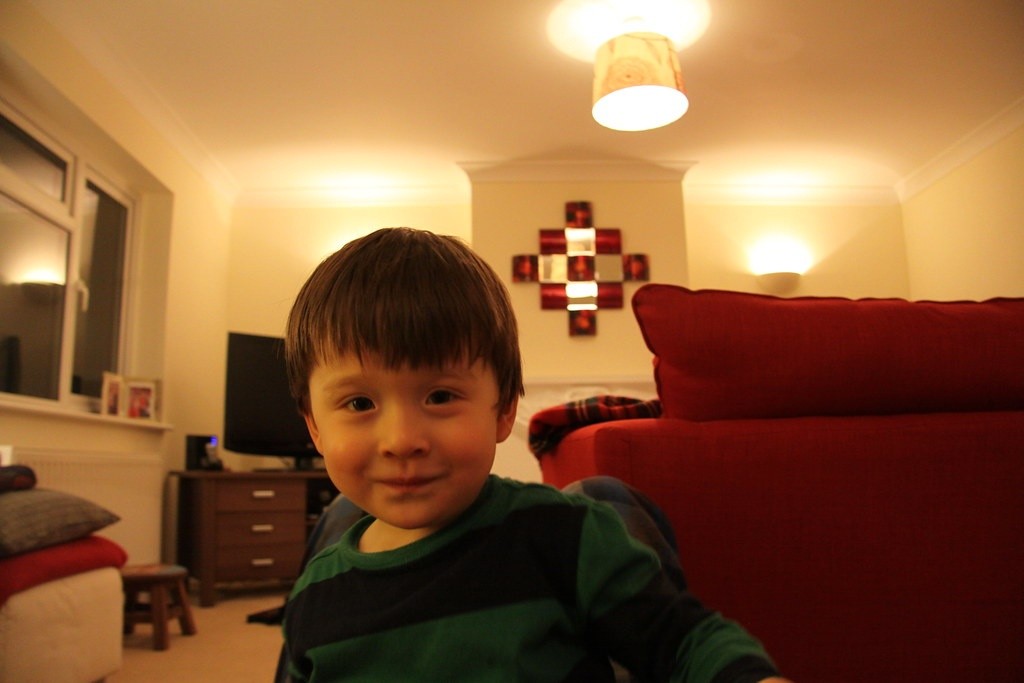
[222,333,326,473]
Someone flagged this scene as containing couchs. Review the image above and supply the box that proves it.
[528,283,1024,683]
[0,534,129,683]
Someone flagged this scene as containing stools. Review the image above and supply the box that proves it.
[120,565,197,651]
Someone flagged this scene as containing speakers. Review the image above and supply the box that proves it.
[185,436,216,472]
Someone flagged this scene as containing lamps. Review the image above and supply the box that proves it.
[591,18,689,133]
[758,271,802,295]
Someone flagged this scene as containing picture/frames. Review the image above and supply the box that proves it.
[99,371,124,417]
[124,375,164,421]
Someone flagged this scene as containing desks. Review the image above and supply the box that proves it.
[167,469,329,609]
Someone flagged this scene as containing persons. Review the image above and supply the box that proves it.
[281,227,792,683]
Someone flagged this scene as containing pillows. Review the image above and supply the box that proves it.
[0,489,121,559]
